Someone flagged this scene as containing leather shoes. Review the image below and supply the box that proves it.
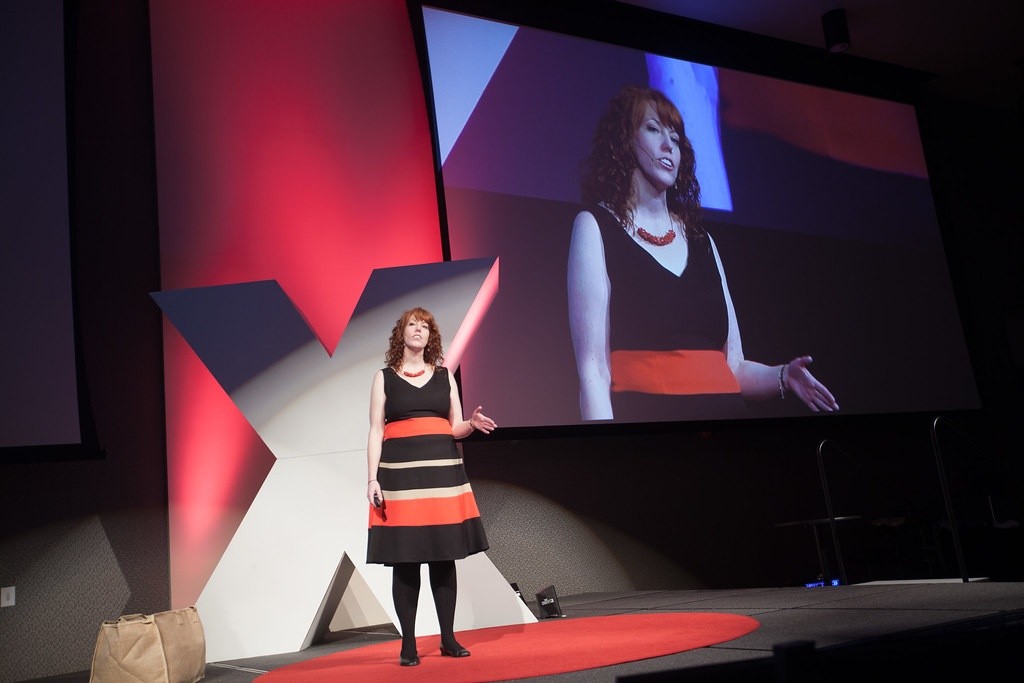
[440,643,470,657]
[399,649,419,666]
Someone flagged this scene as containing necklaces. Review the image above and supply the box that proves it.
[403,369,425,377]
[627,214,675,246]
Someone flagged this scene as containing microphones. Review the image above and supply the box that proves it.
[636,144,655,161]
[405,334,411,338]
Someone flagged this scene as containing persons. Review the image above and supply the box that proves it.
[367,307,498,666]
[567,89,840,419]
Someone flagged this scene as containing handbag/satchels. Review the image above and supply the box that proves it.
[89,606,206,683]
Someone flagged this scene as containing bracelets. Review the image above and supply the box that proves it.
[779,364,788,398]
[368,479,376,484]
[470,420,474,429]
